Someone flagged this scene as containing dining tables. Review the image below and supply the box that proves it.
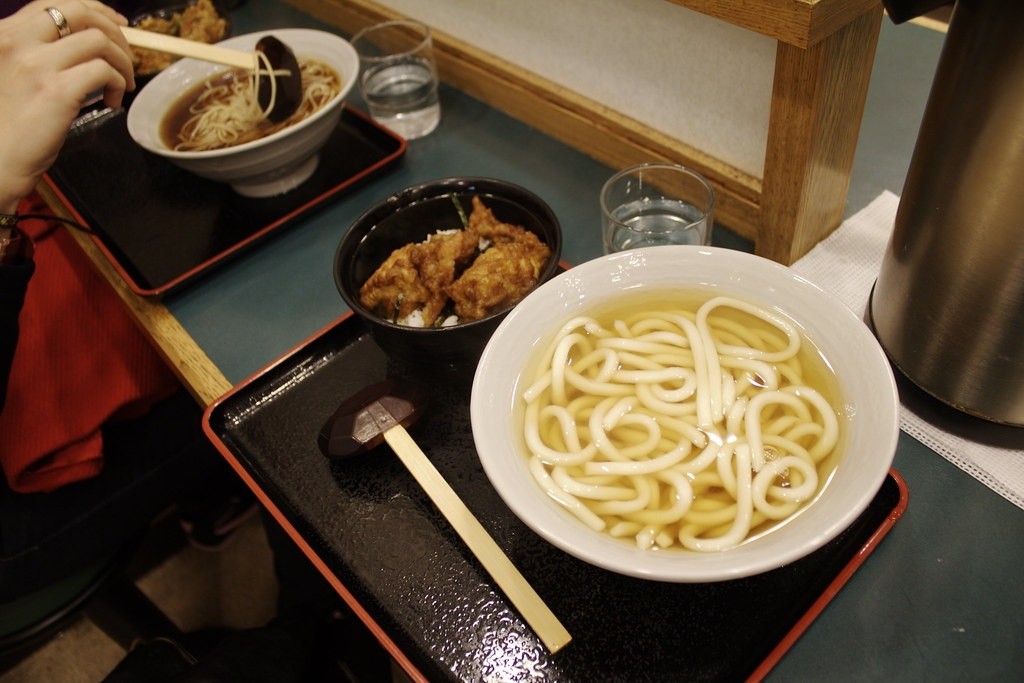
[31,2,1024,683]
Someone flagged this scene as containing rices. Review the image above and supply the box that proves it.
[383,228,490,327]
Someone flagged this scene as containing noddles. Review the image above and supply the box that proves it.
[508,284,847,554]
[160,48,339,151]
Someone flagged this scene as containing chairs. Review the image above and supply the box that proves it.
[0,487,183,676]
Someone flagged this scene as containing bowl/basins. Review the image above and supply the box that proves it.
[127,27,360,197]
[134,25,233,85]
[333,176,545,365]
[470,245,901,582]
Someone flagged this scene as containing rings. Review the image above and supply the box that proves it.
[45,7,72,37]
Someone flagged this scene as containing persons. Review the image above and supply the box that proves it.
[0,0,254,553]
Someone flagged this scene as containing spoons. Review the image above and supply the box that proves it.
[119,26,302,124]
[318,378,572,655]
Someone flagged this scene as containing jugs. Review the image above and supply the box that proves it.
[864,0,1024,449]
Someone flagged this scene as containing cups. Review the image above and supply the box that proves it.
[599,163,715,255]
[350,20,441,139]
